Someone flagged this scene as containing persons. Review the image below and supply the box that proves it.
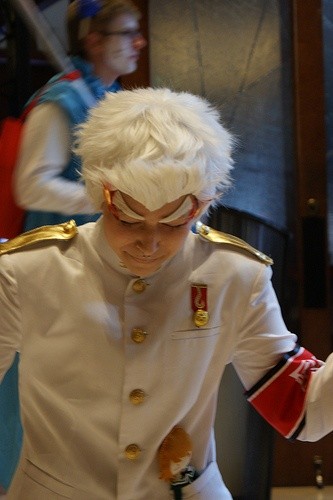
[0,88,333,500]
[14,0,146,231]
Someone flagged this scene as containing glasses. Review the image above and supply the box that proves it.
[103,29,141,40]
